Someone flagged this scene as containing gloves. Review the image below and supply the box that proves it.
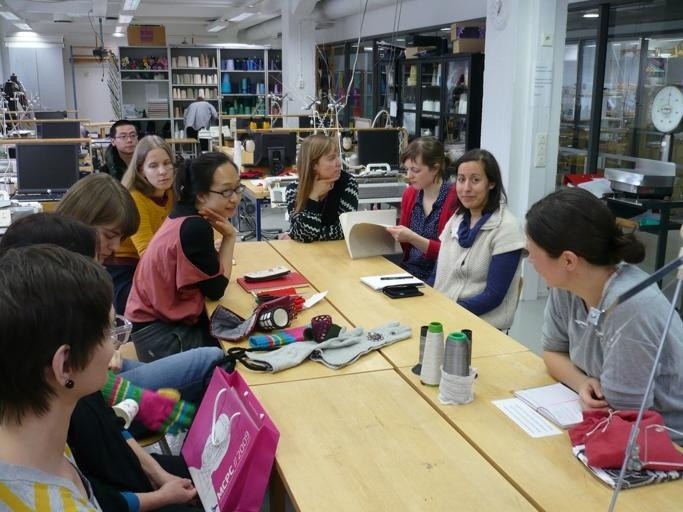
[249,324,340,346]
[313,321,413,371]
[101,369,197,438]
[246,325,363,375]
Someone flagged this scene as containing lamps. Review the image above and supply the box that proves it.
[206,5,255,33]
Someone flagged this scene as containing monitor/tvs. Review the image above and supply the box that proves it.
[37,121,81,152]
[16,143,79,193]
[236,115,399,175]
[34,112,64,119]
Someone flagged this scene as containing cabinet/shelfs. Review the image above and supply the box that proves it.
[117,45,283,164]
[316,17,487,153]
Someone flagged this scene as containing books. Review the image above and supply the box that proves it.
[338,209,403,258]
[513,382,583,429]
[361,273,423,291]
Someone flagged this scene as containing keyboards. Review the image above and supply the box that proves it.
[11,195,65,201]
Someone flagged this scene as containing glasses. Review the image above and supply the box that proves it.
[207,183,246,199]
[101,314,133,346]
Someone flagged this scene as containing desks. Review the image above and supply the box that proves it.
[236,175,401,240]
[84,121,116,138]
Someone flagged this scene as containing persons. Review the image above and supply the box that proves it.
[374,136,461,280]
[0,119,245,512]
[425,148,530,329]
[280,134,358,244]
[524,187,682,445]
[182,97,218,152]
